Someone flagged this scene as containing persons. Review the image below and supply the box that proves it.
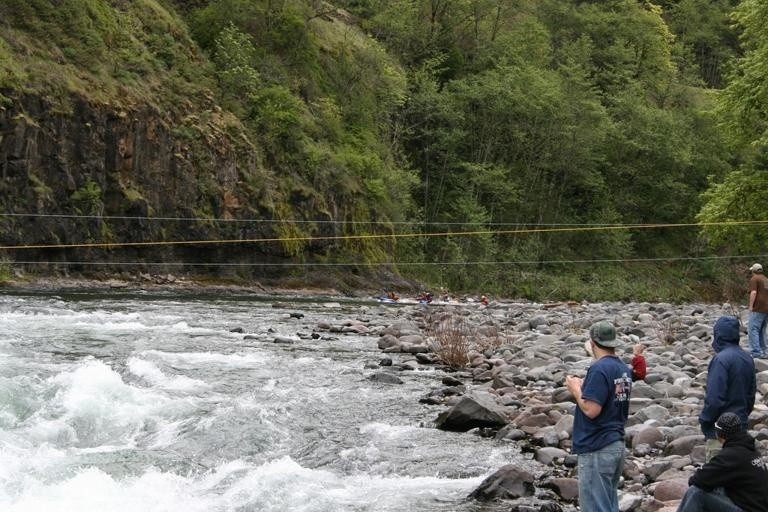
[628,345,646,384]
[561,320,634,512]
[746,263,767,359]
[416,291,432,303]
[447,294,458,303]
[697,316,756,497]
[386,290,399,301]
[670,412,767,512]
[438,289,448,302]
[476,296,489,305]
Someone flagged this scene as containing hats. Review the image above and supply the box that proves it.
[715,412,742,433]
[749,263,763,273]
[590,321,621,348]
